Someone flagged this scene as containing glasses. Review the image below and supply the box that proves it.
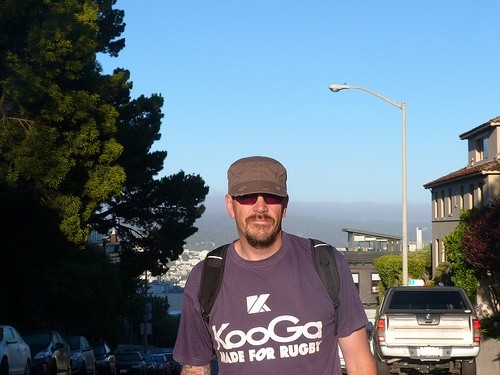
[233,194,283,205]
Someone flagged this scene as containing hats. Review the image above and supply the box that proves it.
[226,156,288,197]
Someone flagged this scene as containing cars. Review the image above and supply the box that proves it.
[373,286,481,375]
[0,325,180,375]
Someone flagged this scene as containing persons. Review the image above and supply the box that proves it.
[173,156,377,375]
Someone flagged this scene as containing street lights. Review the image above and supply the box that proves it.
[328,82,407,287]
